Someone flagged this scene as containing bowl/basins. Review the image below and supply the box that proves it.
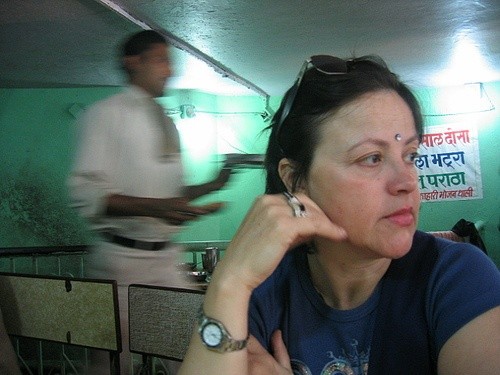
[177,262,196,271]
[188,271,208,282]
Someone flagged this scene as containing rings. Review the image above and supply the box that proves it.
[282,192,306,218]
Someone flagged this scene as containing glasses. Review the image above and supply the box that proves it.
[274,55,391,151]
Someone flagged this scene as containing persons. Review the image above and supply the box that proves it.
[64,29,235,375]
[180,60,500,375]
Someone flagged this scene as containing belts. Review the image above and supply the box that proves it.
[101,231,171,251]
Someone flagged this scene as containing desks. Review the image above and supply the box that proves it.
[181,267,210,289]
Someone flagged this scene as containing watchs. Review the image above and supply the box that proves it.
[198,304,250,353]
